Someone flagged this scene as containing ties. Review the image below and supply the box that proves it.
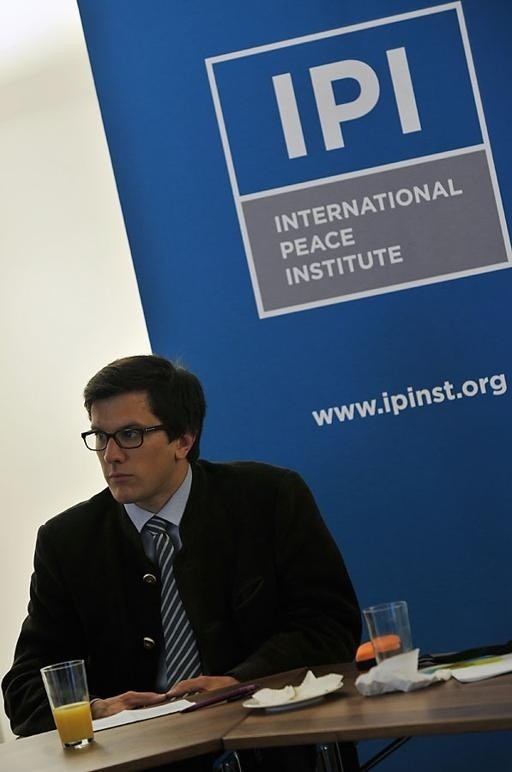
[146,515,204,692]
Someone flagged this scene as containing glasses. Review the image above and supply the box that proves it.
[82,423,164,451]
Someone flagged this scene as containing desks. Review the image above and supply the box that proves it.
[1,663,511,771]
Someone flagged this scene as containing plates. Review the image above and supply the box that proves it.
[241,680,345,713]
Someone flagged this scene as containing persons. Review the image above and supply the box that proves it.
[0,353,367,771]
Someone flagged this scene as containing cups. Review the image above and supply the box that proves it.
[37,658,95,752]
[360,600,414,667]
